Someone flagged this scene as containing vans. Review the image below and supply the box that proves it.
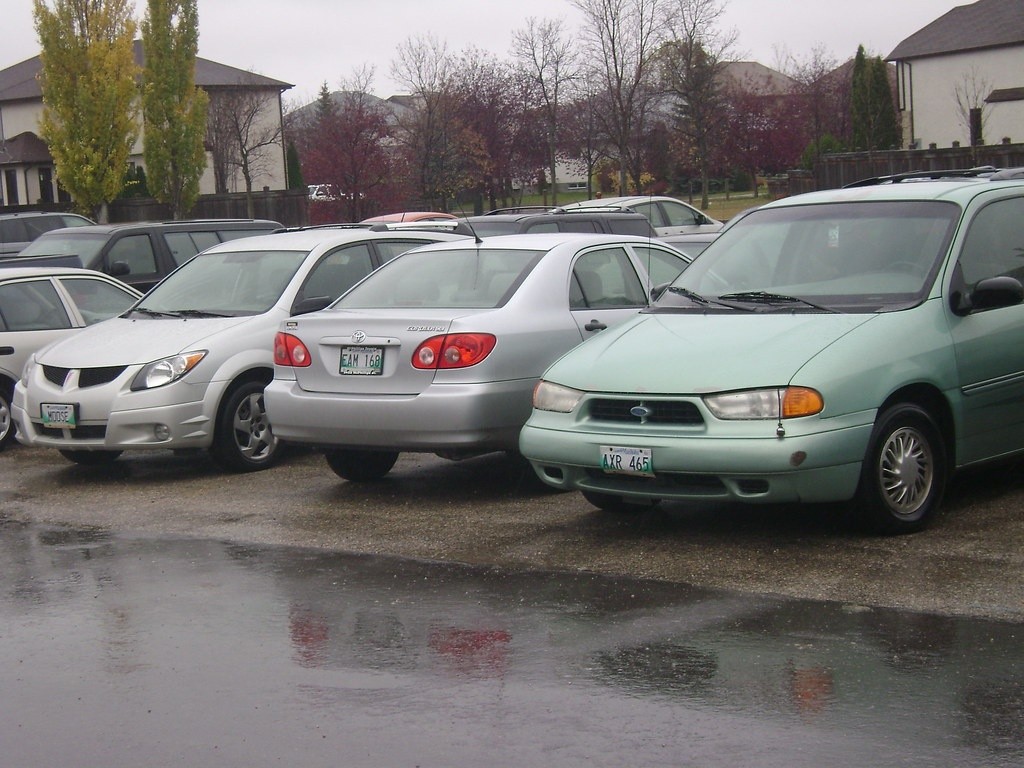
[24,216,287,295]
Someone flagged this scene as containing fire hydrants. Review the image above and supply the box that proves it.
[595,192,602,199]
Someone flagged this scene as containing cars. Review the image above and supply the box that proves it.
[519,165,1024,536]
[0,268,148,453]
[11,219,473,475]
[263,234,729,495]
[0,208,98,258]
[356,196,724,261]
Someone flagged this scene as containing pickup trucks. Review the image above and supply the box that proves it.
[307,183,363,202]
[0,253,83,269]
